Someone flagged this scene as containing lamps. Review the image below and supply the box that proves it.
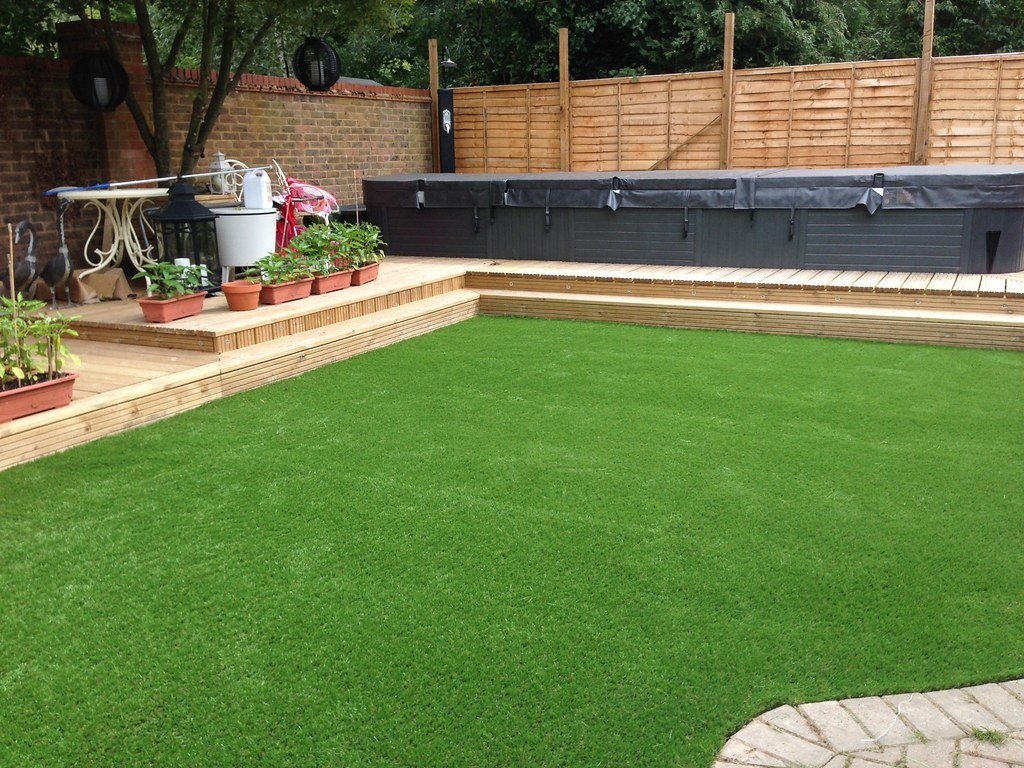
[152,172,222,298]
[441,46,458,88]
[208,150,237,194]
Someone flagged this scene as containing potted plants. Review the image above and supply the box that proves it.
[0,290,81,424]
[243,221,388,312]
[132,261,214,323]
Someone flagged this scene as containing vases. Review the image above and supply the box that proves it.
[221,279,262,311]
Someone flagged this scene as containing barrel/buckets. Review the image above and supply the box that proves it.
[243,170,273,209]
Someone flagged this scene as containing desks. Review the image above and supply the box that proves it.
[57,187,170,297]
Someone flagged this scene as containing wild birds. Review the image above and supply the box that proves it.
[39,197,76,310]
[4,220,41,300]
